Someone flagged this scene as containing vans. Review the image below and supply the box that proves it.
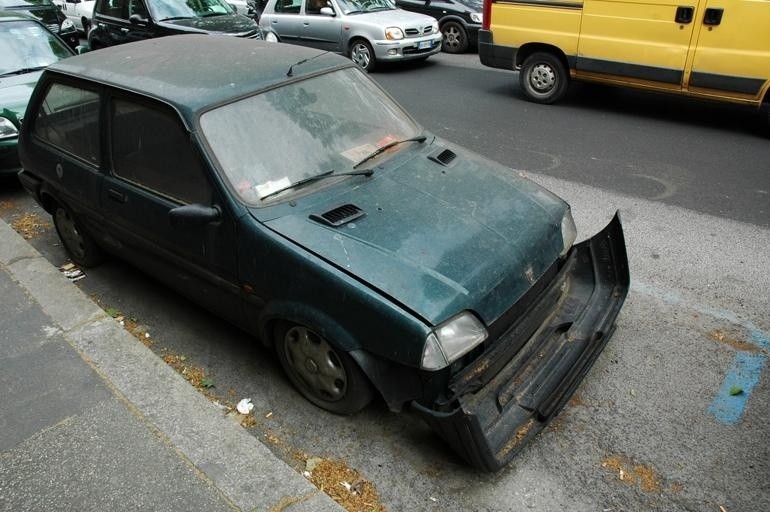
[467,0,769,128]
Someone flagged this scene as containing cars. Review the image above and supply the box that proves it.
[1,10,102,167]
[0,0,81,50]
[394,0,485,55]
[259,0,443,72]
[15,30,632,475]
[54,1,97,36]
[87,1,266,44]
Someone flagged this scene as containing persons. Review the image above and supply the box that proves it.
[309,1,327,14]
